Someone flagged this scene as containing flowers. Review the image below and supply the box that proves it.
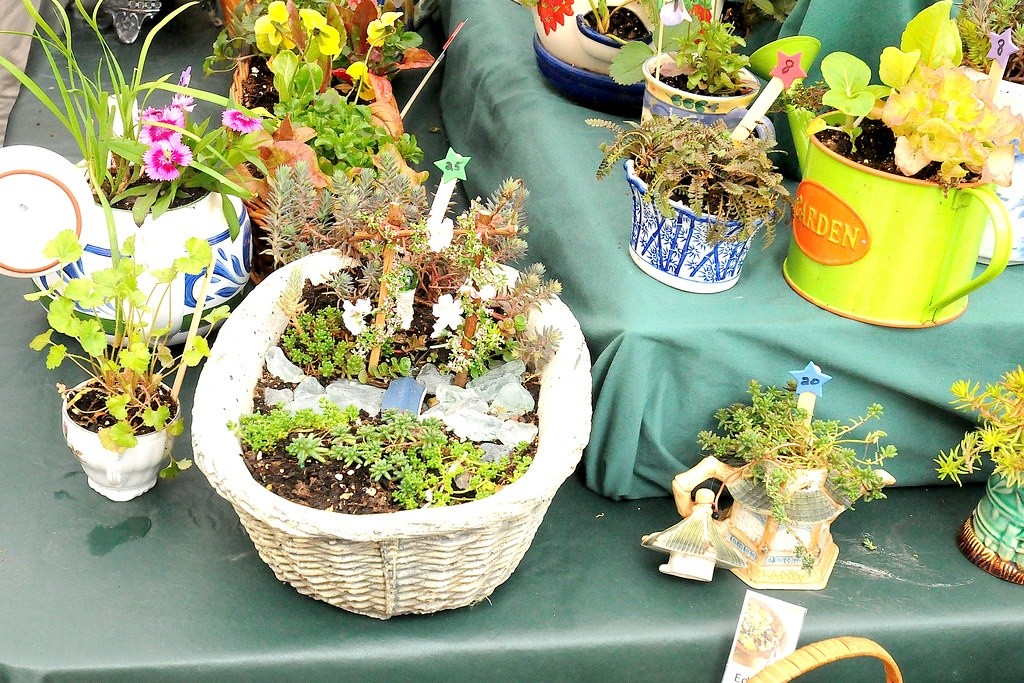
[75,66,249,206]
[208,0,424,144]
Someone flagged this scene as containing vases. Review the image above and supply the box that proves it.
[211,64,421,228]
[28,158,261,352]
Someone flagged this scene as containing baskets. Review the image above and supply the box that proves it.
[190,247,592,621]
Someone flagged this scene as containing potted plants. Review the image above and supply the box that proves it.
[0,0,1024,682]
